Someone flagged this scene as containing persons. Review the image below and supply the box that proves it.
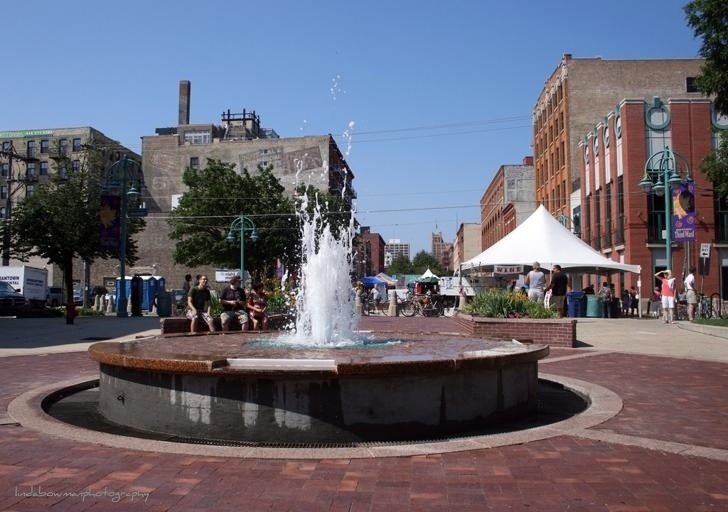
[495,260,698,323]
[354,281,381,317]
[178,273,300,332]
[78,283,108,313]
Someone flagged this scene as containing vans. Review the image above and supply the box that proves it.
[45,286,66,307]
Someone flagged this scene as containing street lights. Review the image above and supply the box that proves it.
[228,211,258,290]
[100,158,146,317]
[637,146,693,320]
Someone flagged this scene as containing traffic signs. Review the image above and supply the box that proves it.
[700,243,711,259]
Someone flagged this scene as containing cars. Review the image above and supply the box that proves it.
[0,280,25,316]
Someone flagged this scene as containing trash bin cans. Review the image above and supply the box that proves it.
[566,291,602,318]
[153,292,172,316]
[116,276,165,309]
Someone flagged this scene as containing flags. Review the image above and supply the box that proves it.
[670,180,695,242]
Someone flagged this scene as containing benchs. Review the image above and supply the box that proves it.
[159,314,293,336]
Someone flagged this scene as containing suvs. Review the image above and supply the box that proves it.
[73,286,107,307]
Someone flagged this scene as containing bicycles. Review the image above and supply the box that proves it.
[362,291,444,317]
[677,292,718,321]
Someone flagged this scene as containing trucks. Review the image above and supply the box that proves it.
[1,266,47,306]
